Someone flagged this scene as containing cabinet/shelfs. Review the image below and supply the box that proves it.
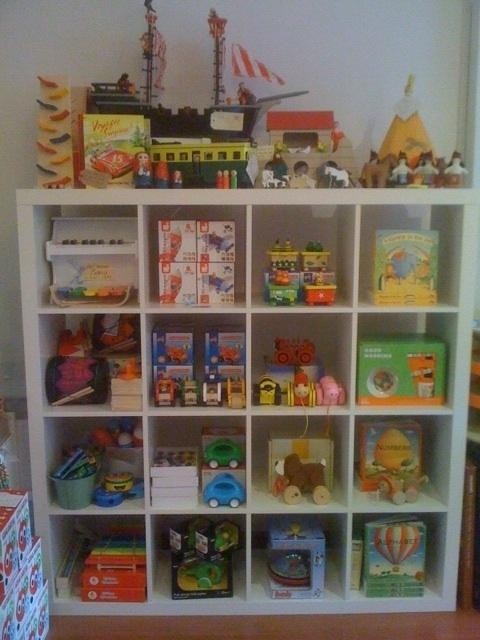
[18,186,480,617]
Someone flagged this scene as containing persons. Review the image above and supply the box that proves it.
[116,73,467,189]
